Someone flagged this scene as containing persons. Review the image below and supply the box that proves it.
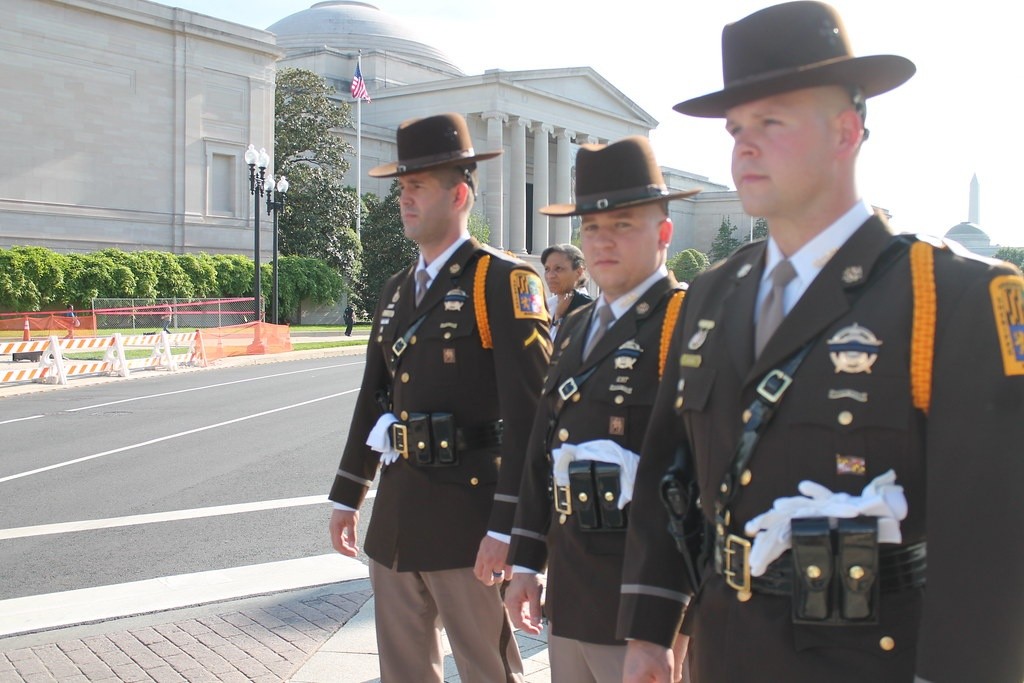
[542,244,595,344]
[63,304,80,339]
[503,137,691,683]
[326,114,553,683]
[161,301,172,334]
[615,1,1024,683]
[343,303,356,336]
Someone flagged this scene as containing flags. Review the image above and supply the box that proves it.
[352,58,372,103]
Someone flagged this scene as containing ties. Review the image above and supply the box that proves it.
[415,270,430,307]
[755,261,799,361]
[583,304,616,361]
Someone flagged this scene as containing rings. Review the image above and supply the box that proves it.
[493,571,502,577]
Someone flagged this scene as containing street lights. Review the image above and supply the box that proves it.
[245,144,269,354]
[265,176,289,352]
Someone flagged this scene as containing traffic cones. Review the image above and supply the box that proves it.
[23,315,32,341]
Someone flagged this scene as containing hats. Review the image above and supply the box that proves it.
[537,135,702,217]
[368,114,503,177]
[672,1,917,118]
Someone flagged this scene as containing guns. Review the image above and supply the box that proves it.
[659,464,701,597]
